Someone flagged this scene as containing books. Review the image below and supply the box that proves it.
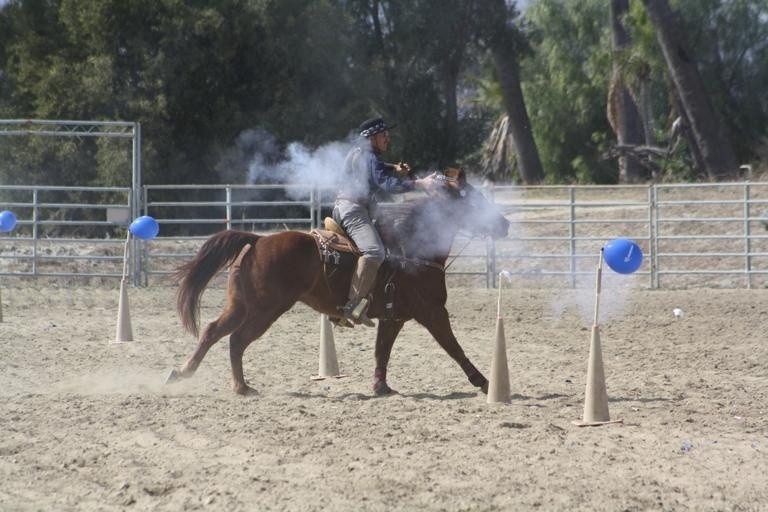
[0,211,18,233]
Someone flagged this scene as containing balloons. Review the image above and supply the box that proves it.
[128,216,161,240]
[602,238,643,275]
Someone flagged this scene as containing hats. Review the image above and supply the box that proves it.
[351,117,397,144]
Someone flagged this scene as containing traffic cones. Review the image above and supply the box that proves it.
[112,277,136,344]
[483,315,514,405]
[566,324,625,426]
[309,311,348,380]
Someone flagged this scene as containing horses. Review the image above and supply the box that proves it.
[163,166,511,399]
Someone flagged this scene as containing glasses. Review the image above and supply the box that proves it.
[376,130,389,138]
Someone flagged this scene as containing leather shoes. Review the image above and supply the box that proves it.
[328,316,354,329]
[344,304,375,328]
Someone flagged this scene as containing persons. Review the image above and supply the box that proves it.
[325,116,445,327]
